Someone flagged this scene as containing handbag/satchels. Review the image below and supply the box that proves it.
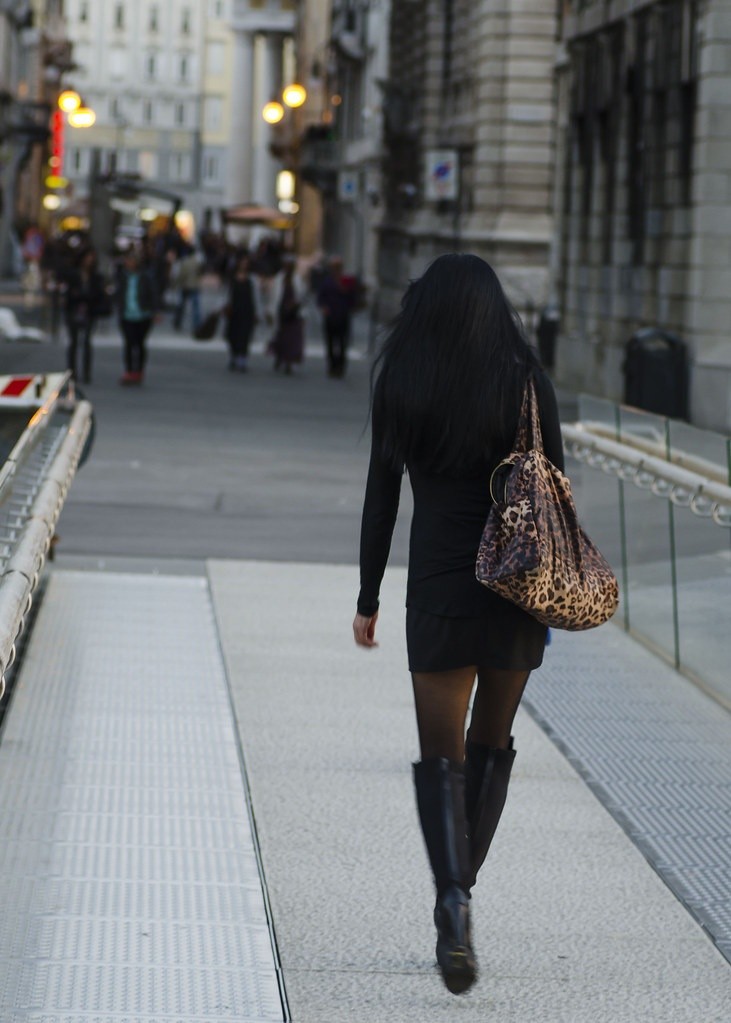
[479,453,615,635]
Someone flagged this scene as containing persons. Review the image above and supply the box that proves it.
[345,253,568,995]
[12,206,360,389]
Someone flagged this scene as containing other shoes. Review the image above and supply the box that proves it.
[118,370,143,387]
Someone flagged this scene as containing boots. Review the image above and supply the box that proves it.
[468,733,517,893]
[410,733,478,993]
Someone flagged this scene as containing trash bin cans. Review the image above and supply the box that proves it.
[618,325,691,426]
